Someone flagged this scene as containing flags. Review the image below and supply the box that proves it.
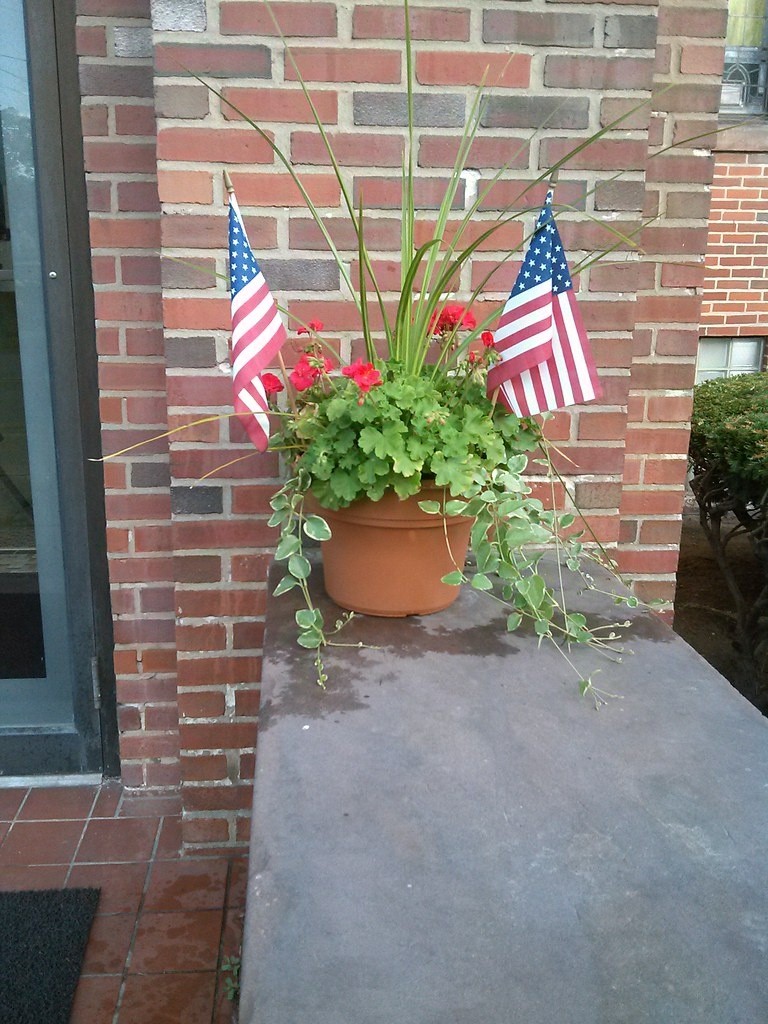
[226,195,289,458]
[484,180,607,422]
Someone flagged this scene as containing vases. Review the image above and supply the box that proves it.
[308,479,476,618]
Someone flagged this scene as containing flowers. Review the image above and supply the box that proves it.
[89,1,763,709]
